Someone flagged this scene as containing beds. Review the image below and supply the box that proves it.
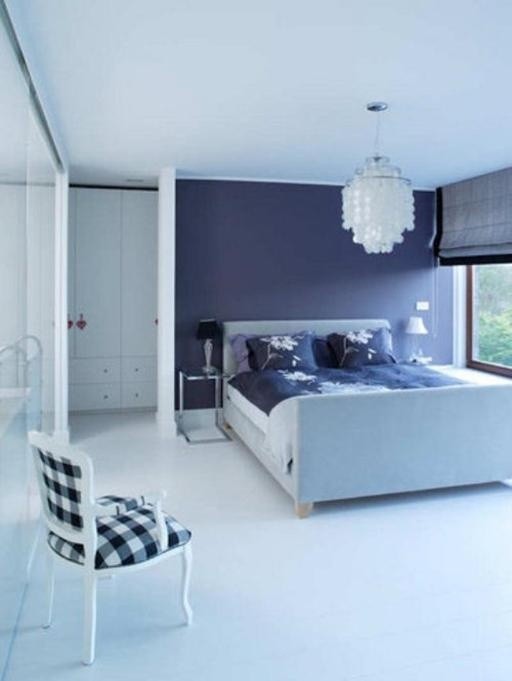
[222,319,512,518]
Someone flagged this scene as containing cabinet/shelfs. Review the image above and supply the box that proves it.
[122,189,159,413]
[67,187,121,416]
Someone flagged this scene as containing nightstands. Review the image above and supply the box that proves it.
[176,367,233,444]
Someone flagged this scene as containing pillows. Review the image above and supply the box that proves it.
[232,328,398,373]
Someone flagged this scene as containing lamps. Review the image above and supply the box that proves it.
[196,319,222,374]
[405,317,428,363]
[341,103,415,254]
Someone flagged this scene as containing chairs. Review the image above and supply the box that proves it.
[28,429,194,665]
